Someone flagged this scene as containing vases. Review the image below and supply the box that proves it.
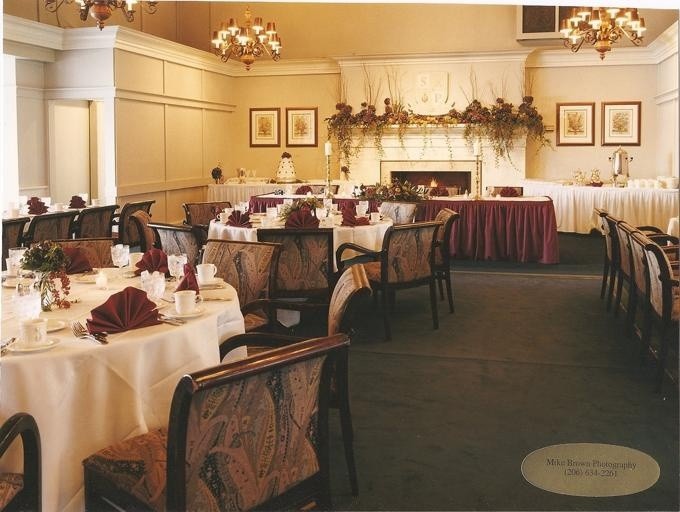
[30,265,56,313]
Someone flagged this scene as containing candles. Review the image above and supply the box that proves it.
[324,140,334,157]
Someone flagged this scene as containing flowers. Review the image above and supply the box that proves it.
[17,237,79,311]
[324,95,553,178]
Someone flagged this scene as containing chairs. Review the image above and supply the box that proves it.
[301,184,523,197]
[2,193,156,269]
[1,411,43,511]
[598,207,678,394]
[146,198,460,338]
[196,239,285,335]
[83,331,351,512]
[216,261,373,497]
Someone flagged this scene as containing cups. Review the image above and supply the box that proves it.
[2,191,102,221]
[212,193,388,229]
[2,245,235,353]
[562,170,678,191]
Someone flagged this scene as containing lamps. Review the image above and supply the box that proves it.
[209,1,283,72]
[41,1,160,32]
[558,7,647,60]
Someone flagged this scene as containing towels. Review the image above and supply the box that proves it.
[83,285,164,339]
[175,258,200,296]
[131,246,174,282]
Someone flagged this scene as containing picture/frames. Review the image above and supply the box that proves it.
[555,100,596,147]
[248,106,282,149]
[285,106,319,148]
[599,100,643,148]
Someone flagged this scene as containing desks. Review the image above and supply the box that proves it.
[205,177,336,212]
[208,212,394,264]
[250,182,563,267]
